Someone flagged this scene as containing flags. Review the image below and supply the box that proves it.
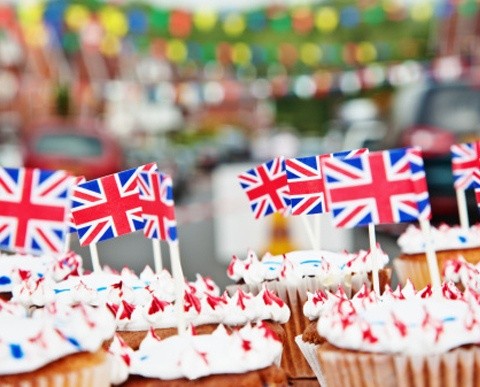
[284,148,369,216]
[71,161,159,248]
[320,147,433,229]
[68,175,85,234]
[471,170,480,208]
[136,170,178,242]
[236,155,293,221]
[449,139,480,190]
[0,166,75,254]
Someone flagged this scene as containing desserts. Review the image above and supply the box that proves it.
[393,224,480,290]
[226,242,393,379]
[294,255,480,387]
[0,251,291,387]
